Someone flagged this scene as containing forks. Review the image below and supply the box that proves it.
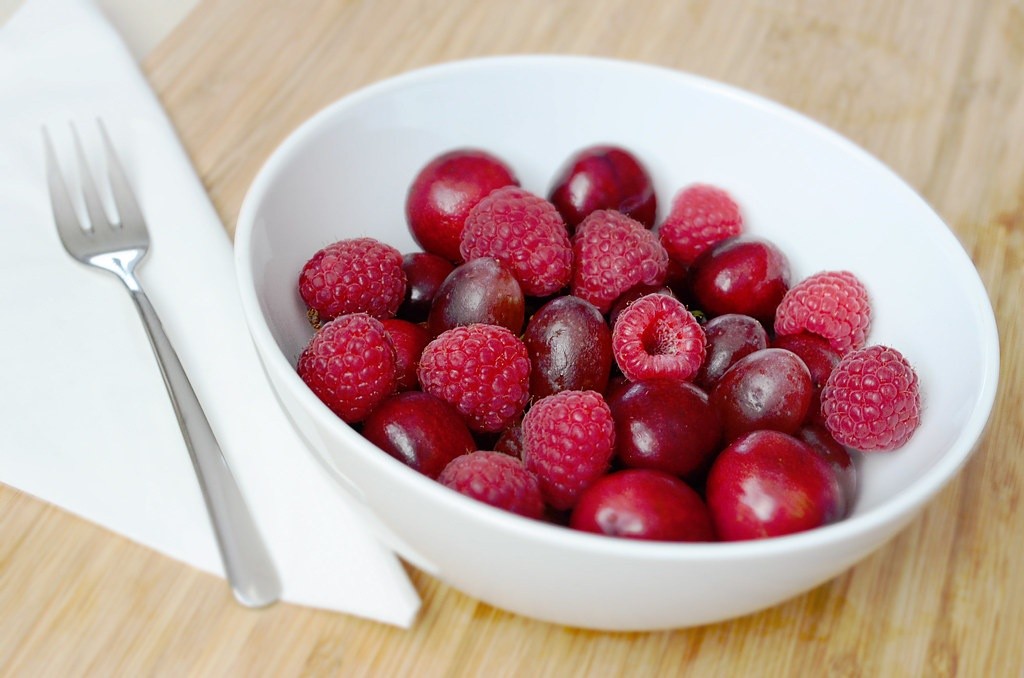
[40,113,281,609]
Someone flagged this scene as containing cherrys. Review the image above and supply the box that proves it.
[374,147,854,542]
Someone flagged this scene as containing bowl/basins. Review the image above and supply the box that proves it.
[234,53,1000,632]
